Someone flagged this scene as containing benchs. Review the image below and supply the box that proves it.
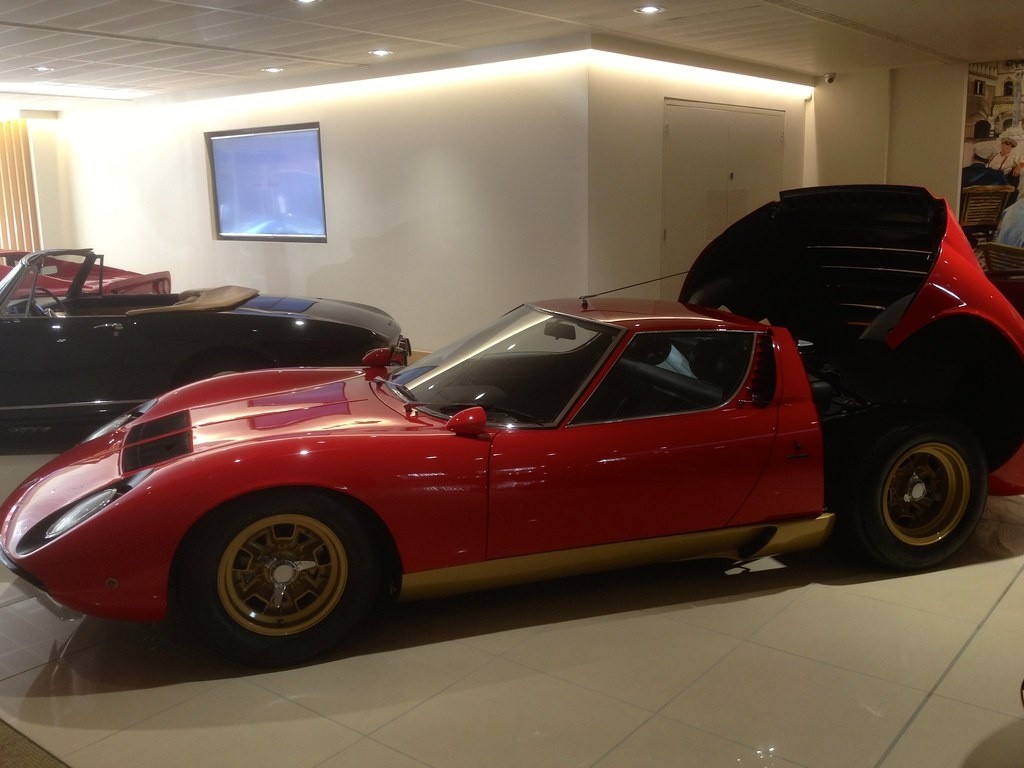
[612,359,724,420]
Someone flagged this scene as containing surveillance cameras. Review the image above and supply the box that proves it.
[824,73,835,84]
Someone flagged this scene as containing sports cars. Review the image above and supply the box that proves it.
[0,246,413,428]
[0,186,1024,670]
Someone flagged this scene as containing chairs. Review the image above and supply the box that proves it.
[961,185,1015,243]
[981,242,1024,314]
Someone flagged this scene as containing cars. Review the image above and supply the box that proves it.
[0,248,174,301]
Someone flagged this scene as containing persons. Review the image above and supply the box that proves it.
[961,134,1024,247]
